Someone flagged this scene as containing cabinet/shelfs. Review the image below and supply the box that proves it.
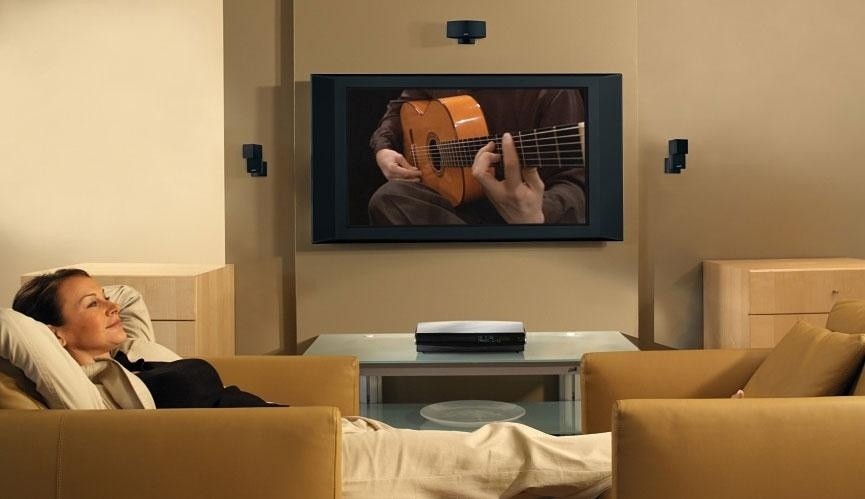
[20,261,234,360]
[701,256,865,351]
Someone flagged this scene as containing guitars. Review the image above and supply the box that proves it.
[399,95,587,208]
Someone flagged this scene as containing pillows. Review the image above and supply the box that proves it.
[727,313,863,399]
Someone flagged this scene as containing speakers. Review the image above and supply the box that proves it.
[664,138,689,174]
[243,144,267,178]
[446,20,487,44]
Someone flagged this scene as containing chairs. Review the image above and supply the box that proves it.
[2,352,357,499]
[580,300,865,499]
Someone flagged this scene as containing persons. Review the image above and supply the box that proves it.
[0,268,744,499]
[362,88,589,226]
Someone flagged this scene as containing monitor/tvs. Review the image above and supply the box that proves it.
[309,72,624,245]
